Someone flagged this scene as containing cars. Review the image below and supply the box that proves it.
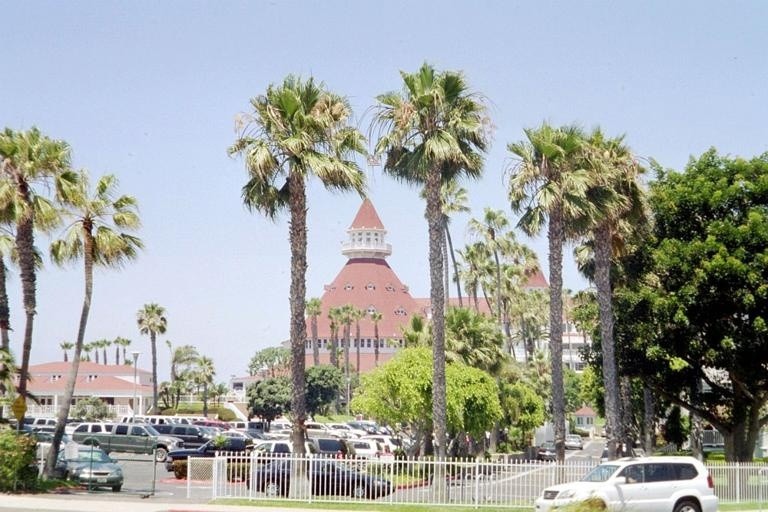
[600,424,607,436]
[537,440,558,460]
[565,434,584,449]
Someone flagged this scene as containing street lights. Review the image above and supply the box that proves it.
[131,351,140,413]
[346,377,352,415]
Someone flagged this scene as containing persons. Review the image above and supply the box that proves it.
[335,448,346,459]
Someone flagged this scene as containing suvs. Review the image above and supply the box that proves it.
[533,455,720,512]
